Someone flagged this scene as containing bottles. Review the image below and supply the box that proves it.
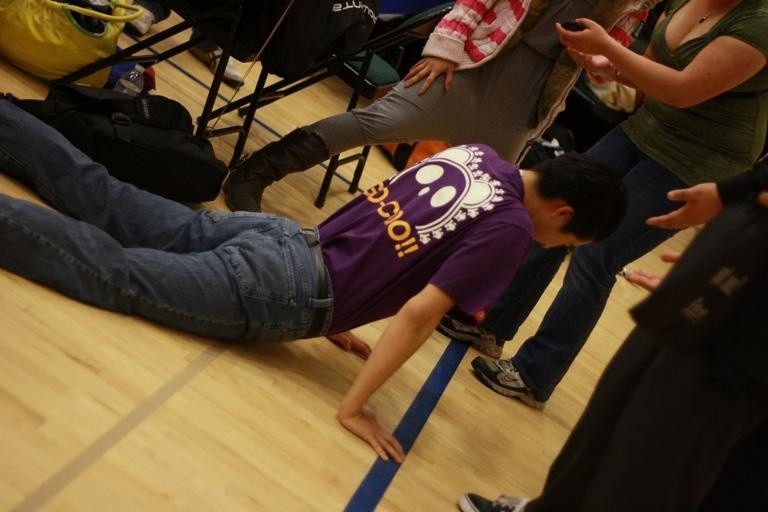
[115,65,145,97]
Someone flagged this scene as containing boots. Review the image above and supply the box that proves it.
[222,125,333,213]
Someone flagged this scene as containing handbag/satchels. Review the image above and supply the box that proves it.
[0,0,143,99]
[0,83,230,204]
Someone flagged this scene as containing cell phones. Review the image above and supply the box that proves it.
[560,20,582,31]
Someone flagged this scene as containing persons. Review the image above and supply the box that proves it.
[126,0,245,86]
[0,98,629,465]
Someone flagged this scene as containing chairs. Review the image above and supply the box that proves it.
[199,1,454,209]
[54,1,380,173]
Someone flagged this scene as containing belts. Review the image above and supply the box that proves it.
[302,229,330,339]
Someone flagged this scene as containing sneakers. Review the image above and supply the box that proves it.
[436,314,506,359]
[457,491,531,512]
[122,3,155,36]
[188,39,245,87]
[471,354,547,411]
